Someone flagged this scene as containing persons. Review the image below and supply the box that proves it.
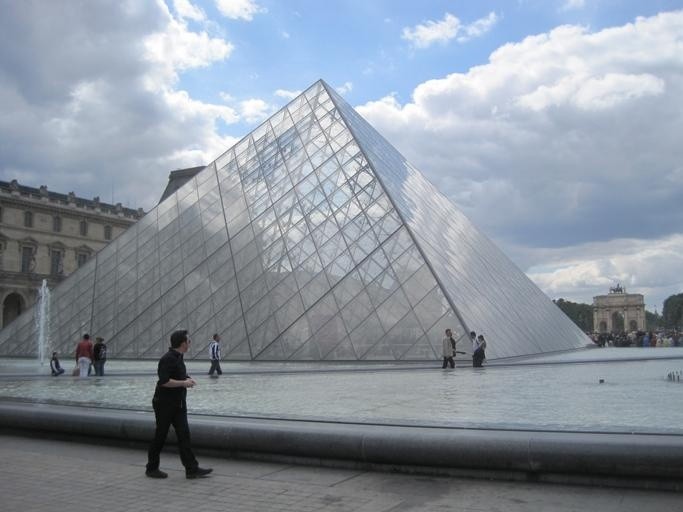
[74,333,94,378]
[470,331,480,353]
[49,351,64,376]
[144,330,213,479]
[589,326,683,347]
[440,328,456,369]
[207,333,222,376]
[91,337,106,376]
[470,334,486,367]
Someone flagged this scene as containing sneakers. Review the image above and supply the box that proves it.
[145,469,167,478]
[186,468,213,479]
